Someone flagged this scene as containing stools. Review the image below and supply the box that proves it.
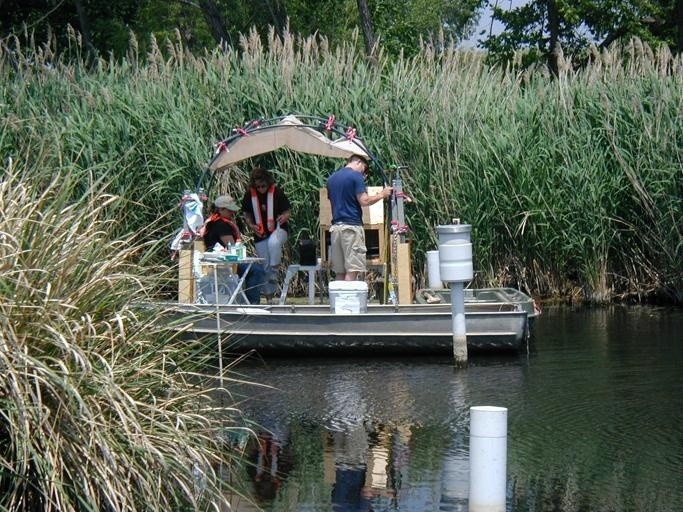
[278,257,327,305]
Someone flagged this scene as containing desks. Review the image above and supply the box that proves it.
[198,256,265,305]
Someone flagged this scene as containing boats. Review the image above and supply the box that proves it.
[124,107,545,365]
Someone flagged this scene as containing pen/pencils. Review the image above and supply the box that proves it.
[384,182,389,203]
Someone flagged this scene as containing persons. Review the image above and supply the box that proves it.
[239,166,292,305]
[323,153,392,280]
[204,194,263,305]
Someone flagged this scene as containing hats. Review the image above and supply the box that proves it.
[215,196,241,212]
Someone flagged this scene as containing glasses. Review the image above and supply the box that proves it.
[255,184,266,188]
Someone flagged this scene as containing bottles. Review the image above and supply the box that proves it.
[235,239,246,260]
